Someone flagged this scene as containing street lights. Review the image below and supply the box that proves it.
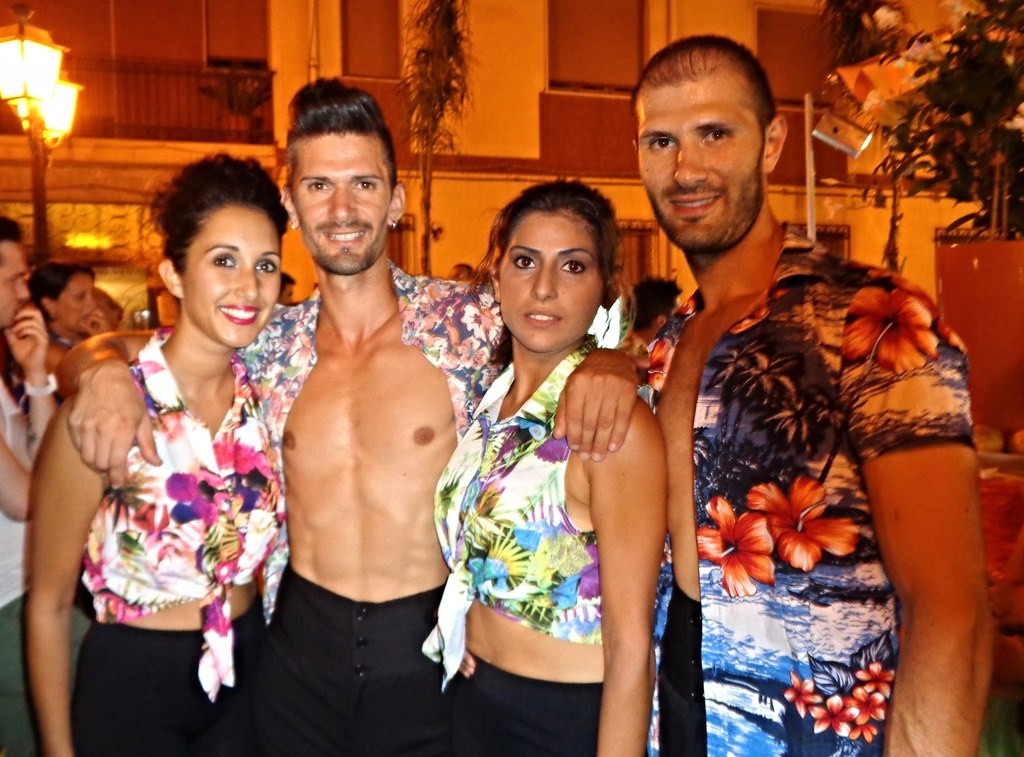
[0,2,86,271]
[804,112,873,240]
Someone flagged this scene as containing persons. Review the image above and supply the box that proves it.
[28,263,111,396]
[57,77,636,757]
[616,276,679,357]
[421,176,666,757]
[635,37,994,757]
[0,215,58,757]
[27,155,290,757]
[447,264,473,281]
[278,273,294,306]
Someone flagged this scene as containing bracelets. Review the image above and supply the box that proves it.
[23,375,57,396]
